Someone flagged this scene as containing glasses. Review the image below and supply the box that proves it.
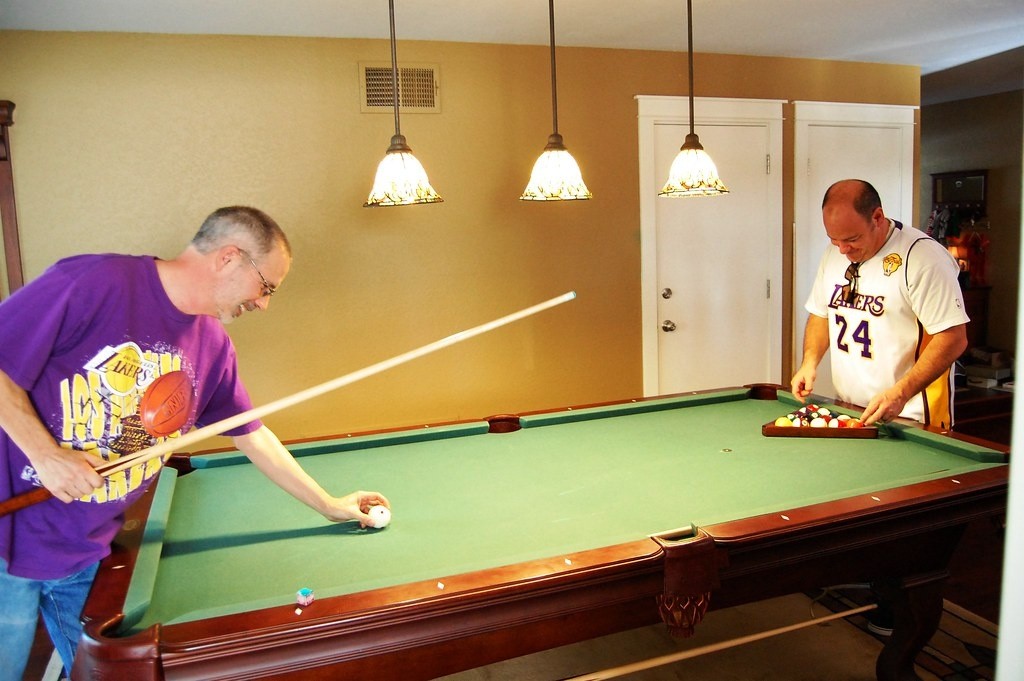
[842,262,861,303]
[239,248,276,297]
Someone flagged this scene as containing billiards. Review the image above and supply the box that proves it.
[366,505,392,528]
[775,403,864,428]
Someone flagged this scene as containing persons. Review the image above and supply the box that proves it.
[791,179,971,636]
[0,206,389,681]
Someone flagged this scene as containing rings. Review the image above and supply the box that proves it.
[882,417,886,421]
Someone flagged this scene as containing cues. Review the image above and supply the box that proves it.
[1,291,579,520]
[566,599,881,681]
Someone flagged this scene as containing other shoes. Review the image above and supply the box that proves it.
[867,607,895,636]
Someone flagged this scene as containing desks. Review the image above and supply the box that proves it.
[76,382,1013,680]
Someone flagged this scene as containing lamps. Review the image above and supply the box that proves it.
[518,0,594,203]
[656,0,730,199]
[363,1,444,208]
